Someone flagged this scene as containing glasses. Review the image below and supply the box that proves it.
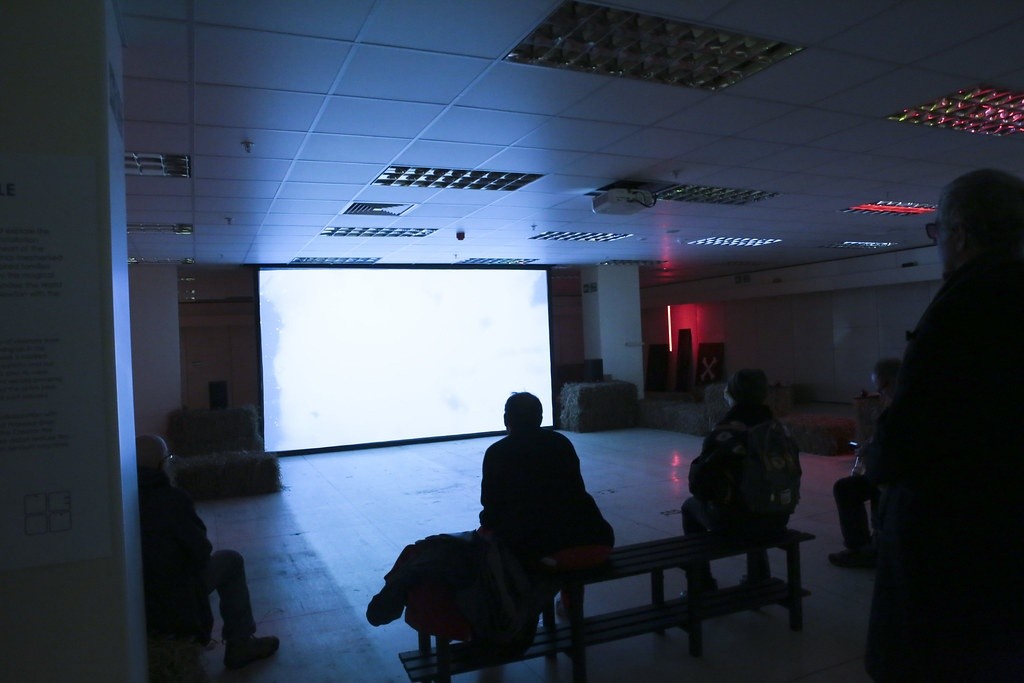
[162,452,175,463]
[925,222,939,239]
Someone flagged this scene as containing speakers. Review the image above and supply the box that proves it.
[584,358,604,383]
[207,379,228,411]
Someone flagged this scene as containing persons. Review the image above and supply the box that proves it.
[136,433,278,669]
[863,167,1024,682]
[680,366,802,606]
[827,356,901,570]
[479,392,614,561]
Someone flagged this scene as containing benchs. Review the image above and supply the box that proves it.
[398,529,818,683]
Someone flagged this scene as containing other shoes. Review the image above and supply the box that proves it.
[828,548,876,568]
[223,634,280,669]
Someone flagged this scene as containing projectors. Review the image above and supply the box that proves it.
[591,188,654,217]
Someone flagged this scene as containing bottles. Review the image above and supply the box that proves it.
[851,455,866,476]
